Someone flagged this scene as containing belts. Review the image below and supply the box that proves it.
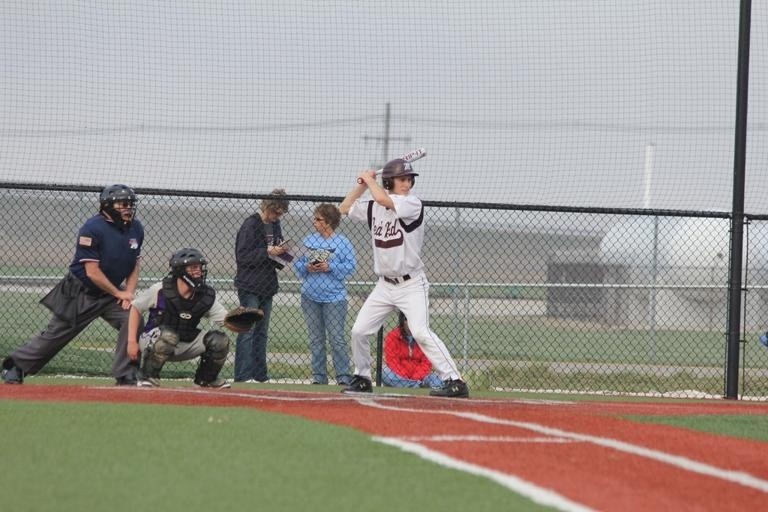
[383,274,411,285]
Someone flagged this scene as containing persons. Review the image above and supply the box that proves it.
[340,157,469,399]
[126,248,264,388]
[293,203,356,385]
[3,185,144,386]
[234,188,290,384]
[382,310,443,388]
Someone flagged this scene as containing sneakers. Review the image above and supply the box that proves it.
[430,379,470,399]
[244,378,278,385]
[115,373,139,386]
[341,377,374,395]
[192,380,232,390]
[1,364,24,384]
[139,377,162,388]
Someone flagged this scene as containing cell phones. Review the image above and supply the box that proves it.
[312,259,319,265]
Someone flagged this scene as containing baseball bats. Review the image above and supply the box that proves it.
[358,147,426,185]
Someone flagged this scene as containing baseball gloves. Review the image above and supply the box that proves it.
[224,307,264,334]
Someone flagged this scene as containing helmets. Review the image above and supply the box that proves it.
[99,184,138,230]
[171,246,210,293]
[381,158,417,191]
